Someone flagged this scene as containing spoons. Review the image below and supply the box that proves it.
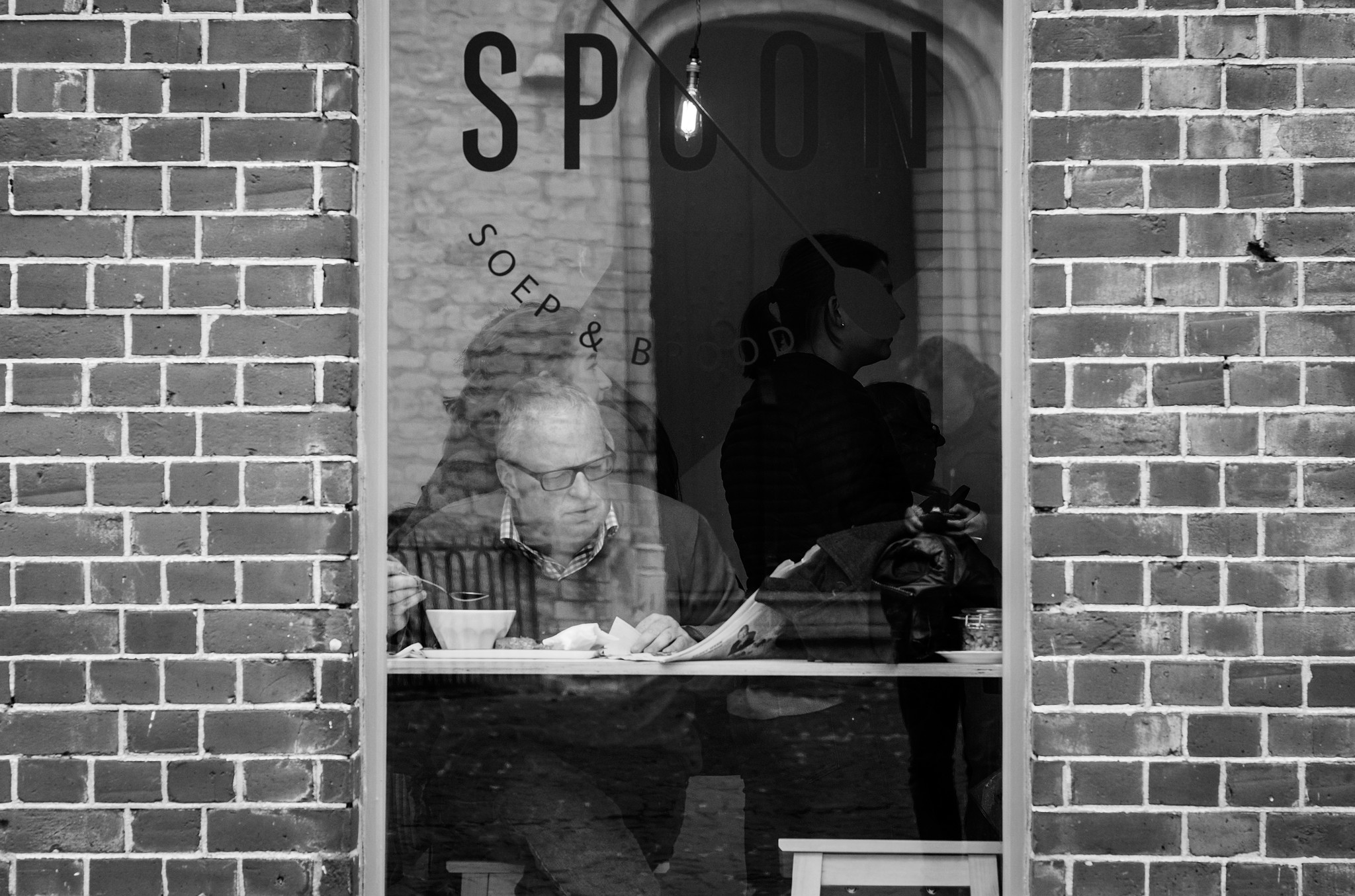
[398,573,490,602]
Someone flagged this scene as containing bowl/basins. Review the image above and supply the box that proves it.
[424,608,517,649]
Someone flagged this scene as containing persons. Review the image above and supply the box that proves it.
[862,382,945,510]
[880,338,1004,896]
[388,304,612,553]
[389,378,752,894]
[717,233,909,589]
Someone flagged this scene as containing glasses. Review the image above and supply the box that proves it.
[493,443,617,492]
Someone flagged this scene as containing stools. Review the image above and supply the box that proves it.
[445,857,668,896]
[775,837,1004,896]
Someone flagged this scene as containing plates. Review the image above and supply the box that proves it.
[935,651,1002,665]
[421,649,601,659]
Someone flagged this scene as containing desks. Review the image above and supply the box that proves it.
[387,655,1000,677]
